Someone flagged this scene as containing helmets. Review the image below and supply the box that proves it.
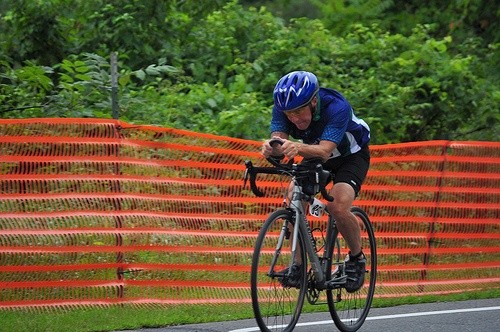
[273,70,319,111]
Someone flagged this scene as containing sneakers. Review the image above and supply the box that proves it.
[288,264,302,284]
[345,252,366,293]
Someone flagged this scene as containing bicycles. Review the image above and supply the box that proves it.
[240,140,376,332]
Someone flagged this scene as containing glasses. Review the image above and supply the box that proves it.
[282,105,311,116]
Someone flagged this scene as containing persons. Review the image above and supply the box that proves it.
[263,70,371,293]
